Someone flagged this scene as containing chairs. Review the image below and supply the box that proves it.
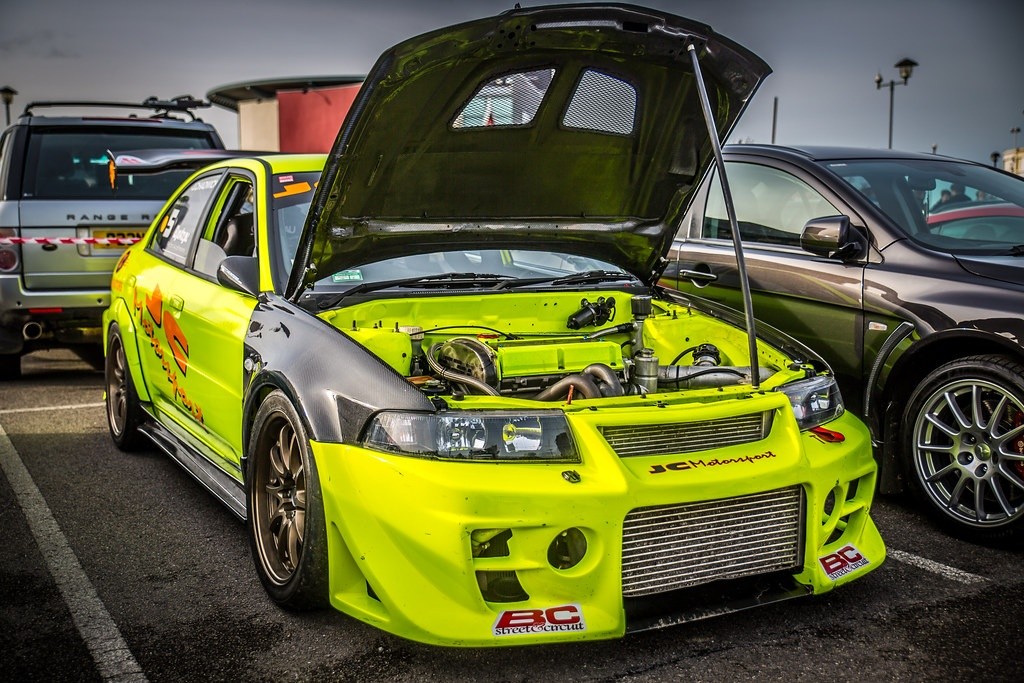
[222,205,308,258]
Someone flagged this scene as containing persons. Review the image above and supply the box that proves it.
[913,189,925,210]
[948,183,971,203]
[932,190,951,212]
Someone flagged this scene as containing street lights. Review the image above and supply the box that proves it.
[874,57,920,152]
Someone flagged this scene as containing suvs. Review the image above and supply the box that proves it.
[0,96,229,382]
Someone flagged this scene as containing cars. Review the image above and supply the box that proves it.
[100,1,889,651]
[474,144,1024,551]
